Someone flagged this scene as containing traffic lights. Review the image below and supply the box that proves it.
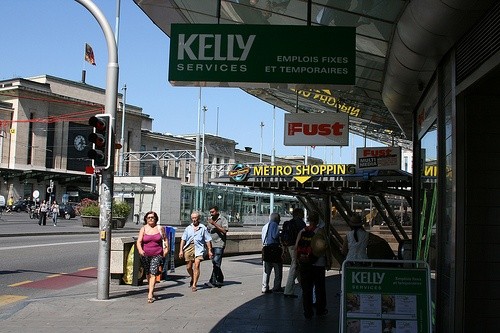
[87,112,112,169]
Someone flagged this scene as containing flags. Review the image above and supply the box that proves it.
[85,43,95,65]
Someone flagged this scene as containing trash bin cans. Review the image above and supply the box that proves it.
[120,237,144,286]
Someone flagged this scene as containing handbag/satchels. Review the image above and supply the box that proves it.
[262,246,283,263]
[281,246,292,265]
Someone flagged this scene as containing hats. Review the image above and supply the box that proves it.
[349,216,362,226]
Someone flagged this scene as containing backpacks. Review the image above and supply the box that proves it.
[281,218,299,246]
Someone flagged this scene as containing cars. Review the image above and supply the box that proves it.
[7,199,40,212]
[45,204,75,220]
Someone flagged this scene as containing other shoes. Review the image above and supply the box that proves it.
[204,282,223,288]
[188,282,192,288]
[284,294,298,298]
[305,316,312,320]
[192,287,197,292]
[262,290,273,293]
[316,315,323,319]
[273,288,284,293]
[158,225,167,253]
[148,299,154,303]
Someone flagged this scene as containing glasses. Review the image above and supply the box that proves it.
[146,216,156,219]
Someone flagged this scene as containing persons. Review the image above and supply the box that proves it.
[179,210,213,292]
[137,212,168,302]
[341,215,370,267]
[205,206,228,288]
[64,202,72,220]
[14,196,23,212]
[38,200,47,225]
[7,196,13,212]
[261,213,285,294]
[283,208,306,298]
[51,201,60,227]
[295,212,332,319]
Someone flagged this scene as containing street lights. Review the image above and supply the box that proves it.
[200,106,209,212]
[259,121,265,163]
